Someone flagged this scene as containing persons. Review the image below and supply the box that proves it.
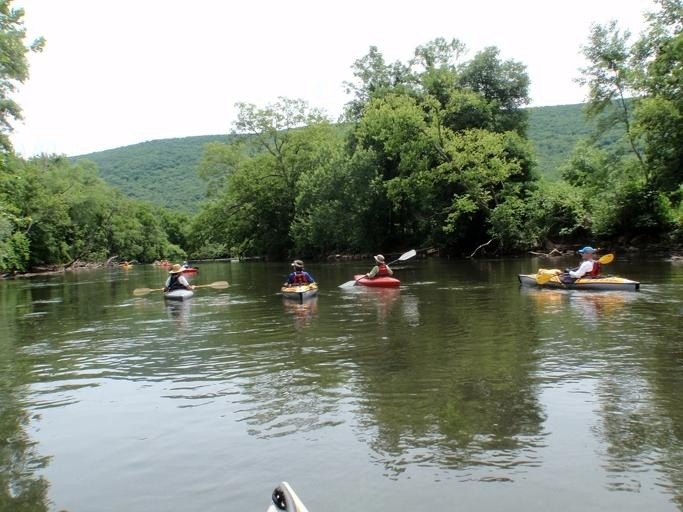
[292,305,314,332]
[168,301,190,333]
[563,245,599,278]
[575,300,603,332]
[164,263,192,291]
[372,295,391,326]
[281,258,313,287]
[364,254,393,280]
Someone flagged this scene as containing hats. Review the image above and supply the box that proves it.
[374,254,385,264]
[579,247,594,253]
[291,260,304,268]
[168,263,182,273]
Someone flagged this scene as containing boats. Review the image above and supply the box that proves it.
[281,281,320,304]
[161,286,196,300]
[282,298,318,330]
[518,272,641,293]
[519,285,639,305]
[150,262,200,274]
[352,274,402,289]
[121,264,134,268]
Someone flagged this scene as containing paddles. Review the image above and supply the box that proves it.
[337,249,417,288]
[132,281,229,295]
[168,267,199,271]
[535,254,615,285]
